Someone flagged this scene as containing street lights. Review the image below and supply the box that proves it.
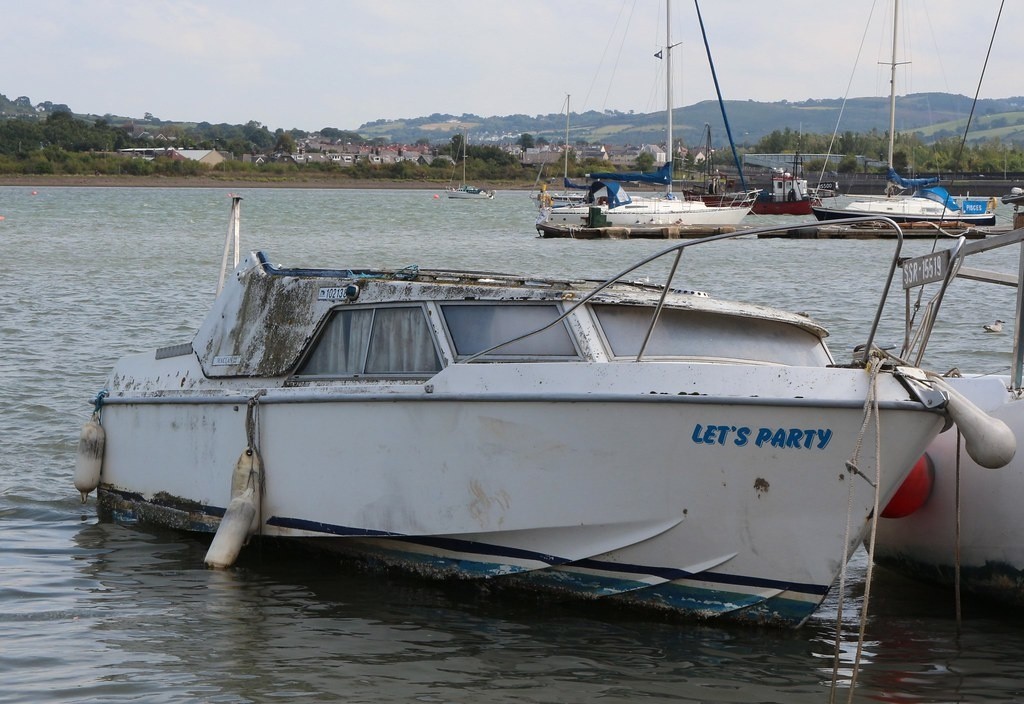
[1004,143,1012,179]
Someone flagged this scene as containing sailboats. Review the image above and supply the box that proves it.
[445,126,496,200]
[682,123,728,202]
[529,95,586,208]
[539,0,763,226]
[809,0,996,226]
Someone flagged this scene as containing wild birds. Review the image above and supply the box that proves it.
[983,320,1005,333]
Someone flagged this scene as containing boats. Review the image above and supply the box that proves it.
[701,150,822,215]
[854,226,1024,598]
[89,195,954,629]
